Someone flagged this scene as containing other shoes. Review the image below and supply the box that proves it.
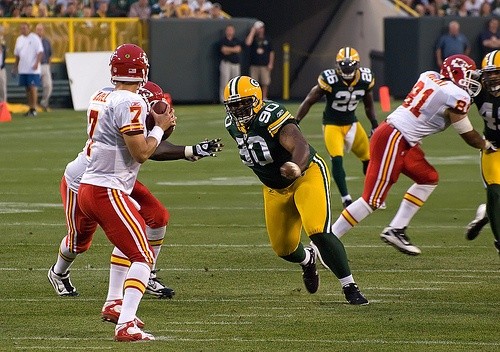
[26,108,37,118]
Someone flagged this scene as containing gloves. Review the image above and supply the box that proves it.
[184,137,222,162]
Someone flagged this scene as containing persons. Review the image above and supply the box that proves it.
[0,0,226,57]
[223,76,370,306]
[398,0,500,17]
[310,54,491,269]
[296,47,387,210]
[48,81,224,297]
[0,26,8,103]
[14,23,44,117]
[478,18,500,64]
[245,21,275,101]
[76,43,178,341]
[436,20,472,67]
[35,24,53,113]
[216,25,242,104]
[466,50,500,255]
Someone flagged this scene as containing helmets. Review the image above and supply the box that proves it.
[110,44,149,87]
[479,49,500,97]
[335,47,360,79]
[223,74,262,127]
[138,80,164,116]
[440,54,476,87]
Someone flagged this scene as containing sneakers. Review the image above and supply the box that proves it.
[341,283,368,306]
[100,300,145,328]
[47,263,77,296]
[494,238,500,255]
[379,227,420,254]
[299,247,318,294]
[113,319,156,342]
[466,205,488,241]
[379,203,385,209]
[145,278,174,298]
[342,200,351,208]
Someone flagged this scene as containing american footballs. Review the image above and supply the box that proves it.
[147,101,174,142]
